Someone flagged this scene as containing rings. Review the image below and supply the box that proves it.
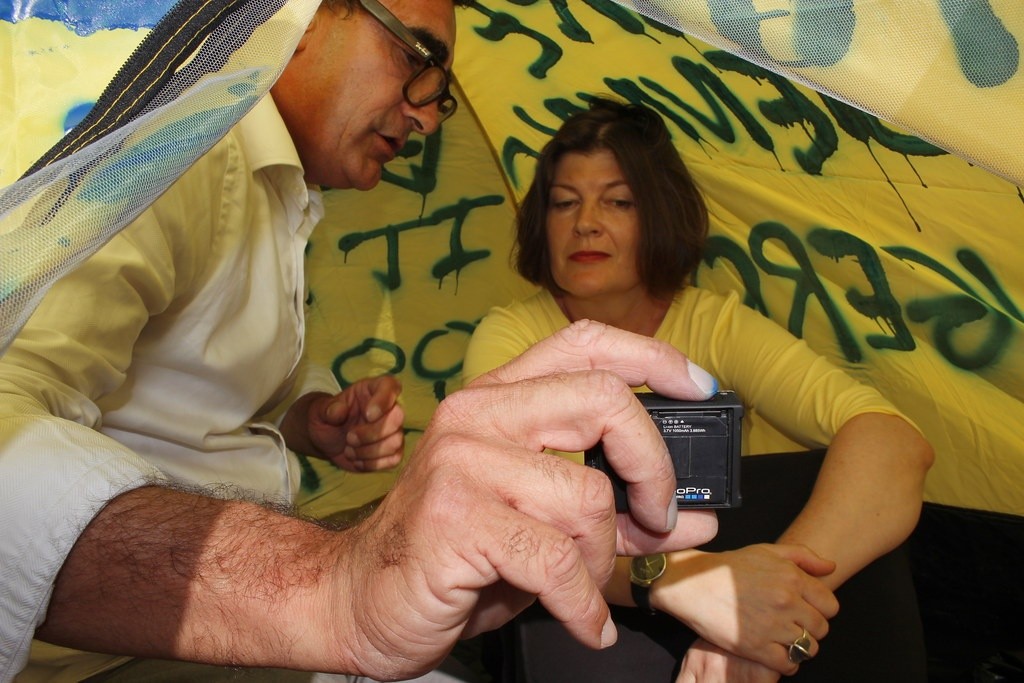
[785,628,814,665]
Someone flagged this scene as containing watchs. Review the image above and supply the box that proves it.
[629,552,667,613]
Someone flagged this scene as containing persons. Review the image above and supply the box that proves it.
[457,99,941,683]
[0,1,723,683]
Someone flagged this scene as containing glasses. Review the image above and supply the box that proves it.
[359,0,458,125]
